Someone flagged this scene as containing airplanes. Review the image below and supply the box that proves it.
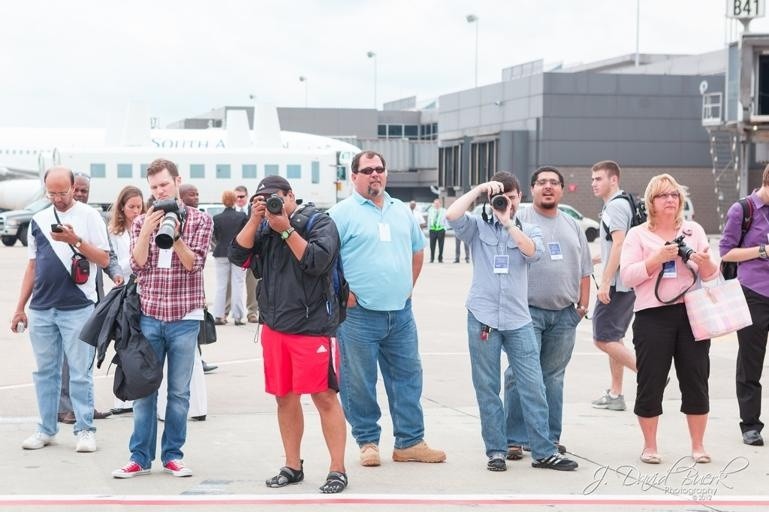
[0,124,383,249]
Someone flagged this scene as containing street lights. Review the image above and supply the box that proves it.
[247,94,261,108]
[462,11,481,90]
[365,48,377,111]
[298,74,308,107]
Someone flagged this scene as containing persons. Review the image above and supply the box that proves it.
[719,164,769,445]
[504,166,594,460]
[590,161,670,410]
[408,199,470,264]
[620,174,721,463]
[10,159,259,477]
[446,171,578,471]
[228,176,348,494]
[324,152,446,465]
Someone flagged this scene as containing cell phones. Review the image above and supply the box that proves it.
[51,224,63,233]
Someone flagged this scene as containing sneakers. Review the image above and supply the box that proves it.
[318,470,348,494]
[507,445,522,459]
[590,387,628,411]
[212,311,259,326]
[358,443,382,466]
[21,431,53,450]
[530,452,579,470]
[162,457,193,477]
[265,461,305,488]
[487,454,508,472]
[392,439,447,464]
[111,459,152,478]
[74,429,96,453]
[428,256,472,265]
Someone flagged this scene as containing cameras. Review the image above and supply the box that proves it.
[264,194,284,214]
[490,191,508,210]
[672,235,696,263]
[153,199,186,249]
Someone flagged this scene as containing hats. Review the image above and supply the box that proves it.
[249,174,294,204]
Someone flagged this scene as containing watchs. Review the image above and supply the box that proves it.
[281,227,294,240]
[504,221,515,231]
[577,302,589,314]
[759,244,768,259]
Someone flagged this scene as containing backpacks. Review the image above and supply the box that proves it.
[601,190,649,246]
[718,196,754,280]
[262,212,349,325]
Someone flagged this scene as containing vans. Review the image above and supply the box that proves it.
[476,203,598,242]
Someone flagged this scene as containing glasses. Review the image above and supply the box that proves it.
[237,194,247,198]
[356,167,386,175]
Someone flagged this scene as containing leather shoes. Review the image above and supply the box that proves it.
[92,408,112,420]
[157,411,207,421]
[57,409,76,424]
[201,358,219,371]
[639,448,662,464]
[691,449,712,464]
[741,429,765,445]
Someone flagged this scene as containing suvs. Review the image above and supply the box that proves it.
[0,195,54,248]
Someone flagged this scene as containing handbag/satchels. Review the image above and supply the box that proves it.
[69,251,91,285]
[195,304,217,346]
[681,267,754,343]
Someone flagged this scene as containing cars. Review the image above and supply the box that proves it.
[405,201,432,228]
